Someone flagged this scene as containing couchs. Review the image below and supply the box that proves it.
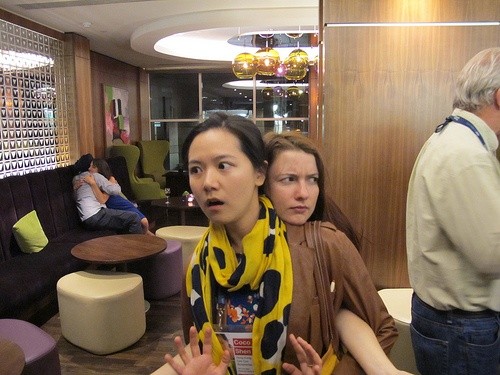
[0,156,160,319]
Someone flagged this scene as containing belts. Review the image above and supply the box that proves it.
[454,309,495,319]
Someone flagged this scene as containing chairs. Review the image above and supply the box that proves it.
[111,137,170,201]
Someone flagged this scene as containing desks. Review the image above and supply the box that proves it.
[72,234,167,313]
[152,193,201,226]
[161,165,189,194]
[0,338,25,375]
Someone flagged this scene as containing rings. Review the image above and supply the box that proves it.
[308,364,312,366]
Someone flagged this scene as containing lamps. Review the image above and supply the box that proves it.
[229,24,308,82]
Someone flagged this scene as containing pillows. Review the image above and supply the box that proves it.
[12,210,49,253]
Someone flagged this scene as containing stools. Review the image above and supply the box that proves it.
[155,226,209,278]
[377,288,420,375]
[0,319,61,375]
[128,239,182,301]
[57,268,146,355]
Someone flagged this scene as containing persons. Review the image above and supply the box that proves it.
[71,153,155,237]
[148,110,413,375]
[405,48,500,375]
[165,130,364,375]
